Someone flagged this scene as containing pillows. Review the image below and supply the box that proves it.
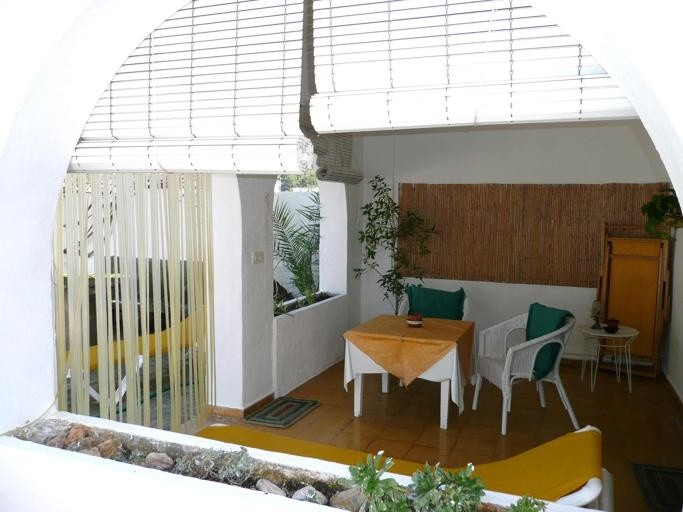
[405,286,465,320]
[526,303,576,381]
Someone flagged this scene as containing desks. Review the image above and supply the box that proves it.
[343,315,475,430]
[576,322,640,394]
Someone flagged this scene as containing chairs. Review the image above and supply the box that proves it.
[472,310,581,435]
[191,421,613,512]
[398,286,468,387]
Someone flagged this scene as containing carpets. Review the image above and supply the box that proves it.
[631,462,683,511]
[244,396,321,429]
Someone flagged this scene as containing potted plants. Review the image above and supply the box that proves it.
[640,182,683,243]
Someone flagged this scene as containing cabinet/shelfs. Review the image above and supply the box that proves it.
[596,223,672,382]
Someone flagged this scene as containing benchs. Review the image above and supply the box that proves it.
[61,302,205,410]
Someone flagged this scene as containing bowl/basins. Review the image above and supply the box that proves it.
[604,326,618,333]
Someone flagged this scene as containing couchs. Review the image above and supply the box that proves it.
[99,256,204,334]
